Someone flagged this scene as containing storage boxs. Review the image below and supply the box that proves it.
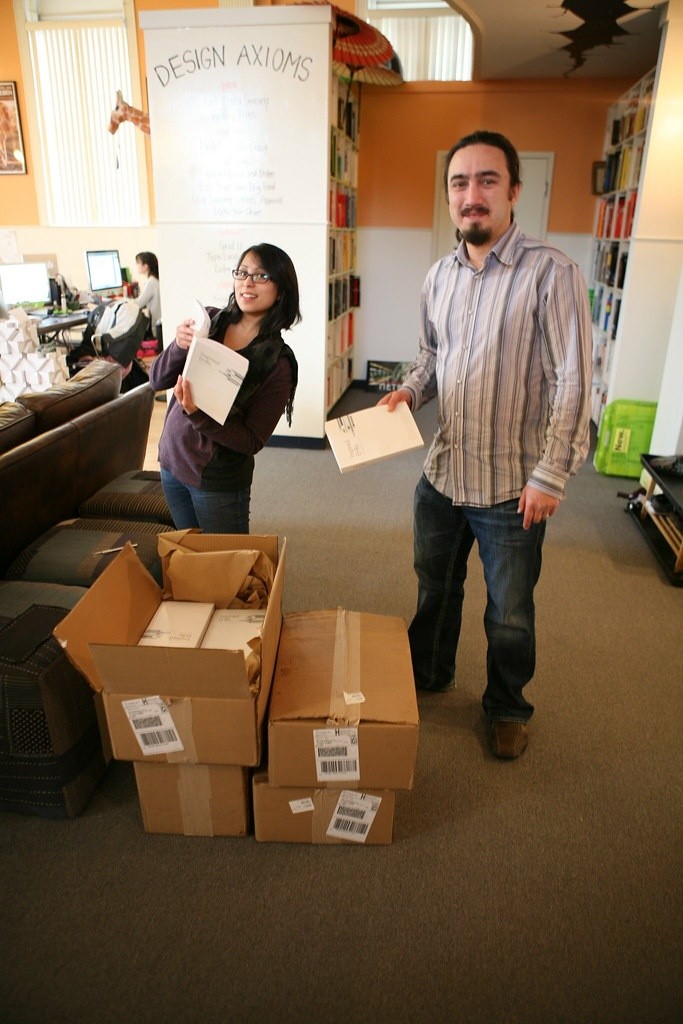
[366,360,419,393]
[592,400,657,479]
[53,528,420,845]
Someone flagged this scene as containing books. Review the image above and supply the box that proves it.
[137,601,217,649]
[324,401,425,473]
[587,74,656,424]
[327,80,362,417]
[182,301,250,425]
[200,610,267,658]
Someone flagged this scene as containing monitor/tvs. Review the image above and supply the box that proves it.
[85,249,124,297]
[0,260,52,305]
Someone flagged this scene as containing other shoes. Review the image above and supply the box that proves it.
[414,674,455,691]
[490,718,528,760]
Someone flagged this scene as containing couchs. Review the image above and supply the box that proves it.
[0,296,157,564]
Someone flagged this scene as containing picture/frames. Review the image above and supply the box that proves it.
[0,81,27,176]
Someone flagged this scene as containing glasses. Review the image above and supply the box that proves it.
[232,270,270,284]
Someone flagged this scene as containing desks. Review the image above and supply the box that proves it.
[27,311,88,350]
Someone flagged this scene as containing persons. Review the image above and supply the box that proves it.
[122,252,161,339]
[375,128,595,760]
[149,243,297,533]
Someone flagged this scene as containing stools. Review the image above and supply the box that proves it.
[81,469,176,527]
[0,579,101,820]
[11,516,177,592]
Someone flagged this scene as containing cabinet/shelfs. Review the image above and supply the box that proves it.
[586,65,683,434]
[628,452,683,587]
[326,67,359,417]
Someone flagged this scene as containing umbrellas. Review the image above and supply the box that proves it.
[333,7,404,87]
